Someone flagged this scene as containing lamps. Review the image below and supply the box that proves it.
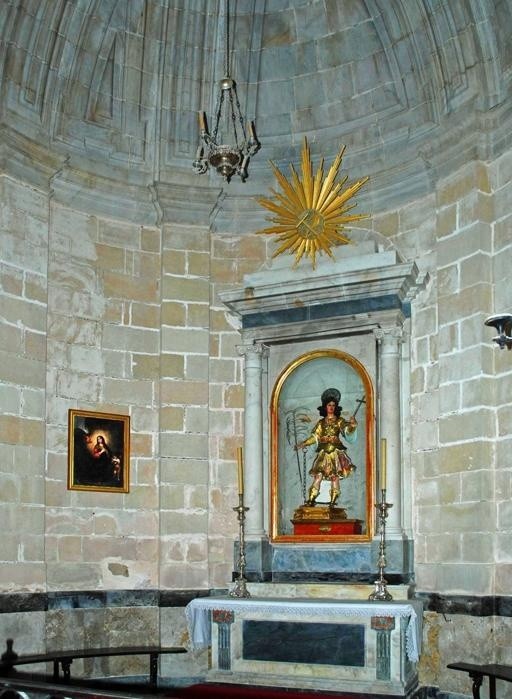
[484,314,512,351]
[190,1,260,183]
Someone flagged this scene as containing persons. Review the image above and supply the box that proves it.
[293,387,359,509]
[73,426,122,484]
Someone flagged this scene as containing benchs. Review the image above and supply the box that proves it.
[8,647,188,690]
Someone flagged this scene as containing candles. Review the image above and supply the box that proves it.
[379,439,387,488]
[237,446,244,494]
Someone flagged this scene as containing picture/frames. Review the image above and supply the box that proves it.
[67,409,133,494]
